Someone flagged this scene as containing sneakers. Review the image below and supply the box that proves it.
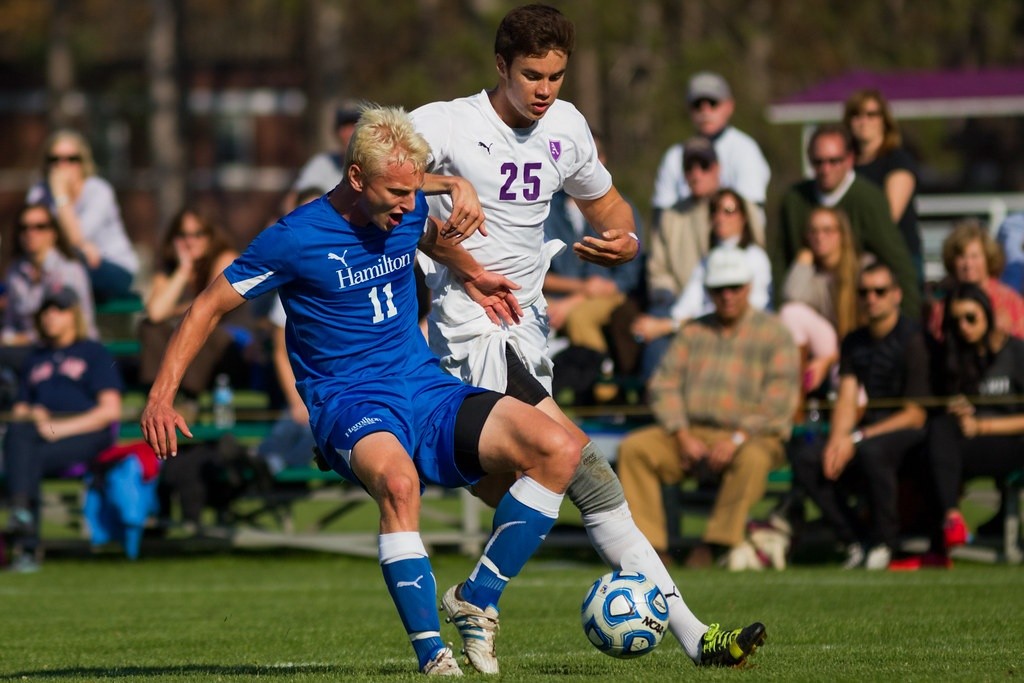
[695,623,766,667]
[439,582,503,675]
[422,647,462,675]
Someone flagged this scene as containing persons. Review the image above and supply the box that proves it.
[1,98,374,572]
[140,109,582,677]
[407,4,767,669]
[543,67,1024,573]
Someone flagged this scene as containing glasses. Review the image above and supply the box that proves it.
[692,96,730,111]
[709,285,751,297]
[951,311,984,326]
[809,150,851,168]
[858,283,899,299]
[682,157,714,172]
[46,151,84,166]
[178,228,212,242]
[19,220,55,234]
[710,206,740,217]
[852,111,881,121]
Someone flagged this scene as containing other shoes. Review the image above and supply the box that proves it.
[893,553,955,570]
[942,512,969,545]
[843,539,895,573]
[658,550,675,569]
[690,543,729,570]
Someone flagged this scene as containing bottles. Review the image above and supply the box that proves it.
[214,374,235,430]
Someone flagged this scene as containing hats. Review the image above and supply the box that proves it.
[37,282,79,312]
[687,71,732,99]
[703,249,753,289]
[681,138,716,166]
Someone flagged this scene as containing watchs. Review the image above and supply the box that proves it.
[852,428,864,448]
[731,432,746,447]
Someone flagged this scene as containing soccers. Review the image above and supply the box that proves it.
[582,570,669,659]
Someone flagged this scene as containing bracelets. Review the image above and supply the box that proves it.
[618,232,640,267]
